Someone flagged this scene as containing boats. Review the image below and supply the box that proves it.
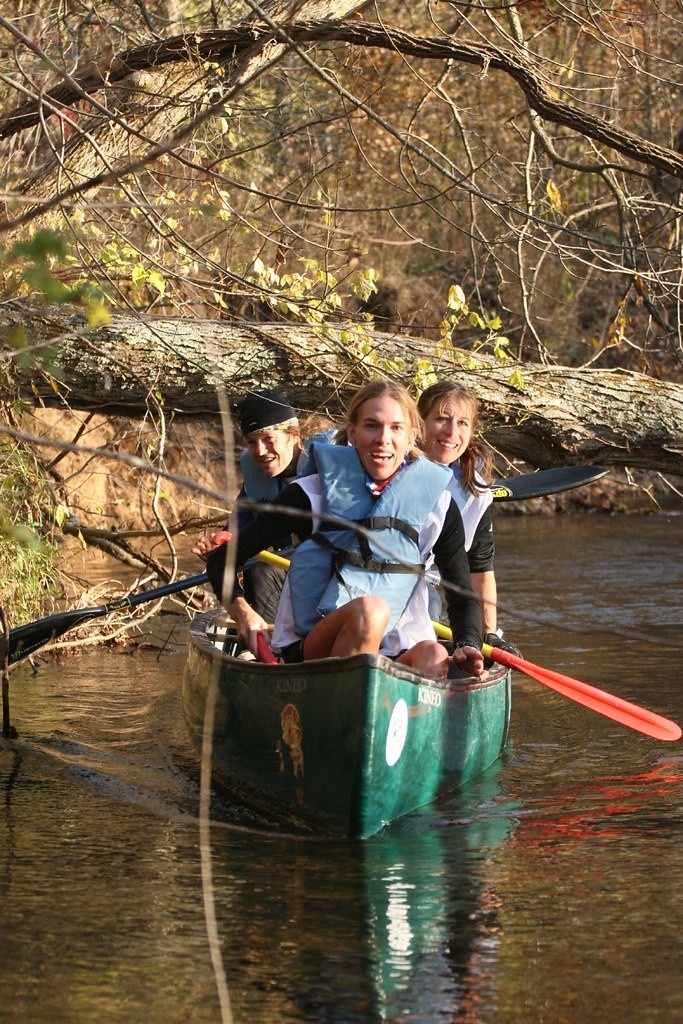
[180,604,532,843]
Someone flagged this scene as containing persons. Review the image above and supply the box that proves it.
[410,379,525,670]
[208,379,484,682]
[222,390,355,665]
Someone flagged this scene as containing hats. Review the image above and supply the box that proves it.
[237,389,295,434]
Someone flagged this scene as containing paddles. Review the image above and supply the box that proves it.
[0,463,609,670]
[208,530,682,742]
